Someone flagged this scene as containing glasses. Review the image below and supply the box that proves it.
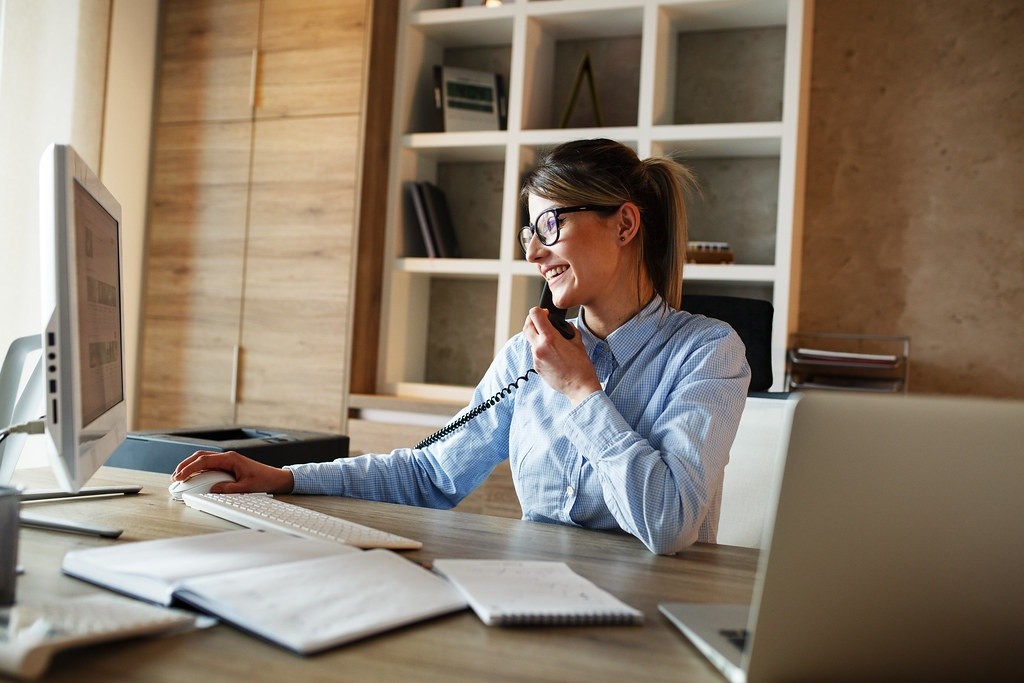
[518,205,620,255]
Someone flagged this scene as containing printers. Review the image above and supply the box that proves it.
[104,424,350,478]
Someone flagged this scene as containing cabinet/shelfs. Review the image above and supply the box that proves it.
[136,0,814,521]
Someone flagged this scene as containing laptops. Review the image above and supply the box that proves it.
[658,391,1024,683]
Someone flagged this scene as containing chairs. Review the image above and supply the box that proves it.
[679,295,798,549]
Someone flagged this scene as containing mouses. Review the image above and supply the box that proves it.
[169,470,236,499]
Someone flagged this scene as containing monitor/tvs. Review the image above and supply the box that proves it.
[0,144,143,540]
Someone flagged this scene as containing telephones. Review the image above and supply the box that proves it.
[536,280,577,341]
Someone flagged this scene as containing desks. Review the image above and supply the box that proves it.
[0,462,760,683]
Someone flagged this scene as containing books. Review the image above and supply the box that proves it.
[408,181,460,260]
[59,528,648,659]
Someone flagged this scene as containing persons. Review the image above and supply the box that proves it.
[170,137,752,556]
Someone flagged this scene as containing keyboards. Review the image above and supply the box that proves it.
[182,493,423,550]
